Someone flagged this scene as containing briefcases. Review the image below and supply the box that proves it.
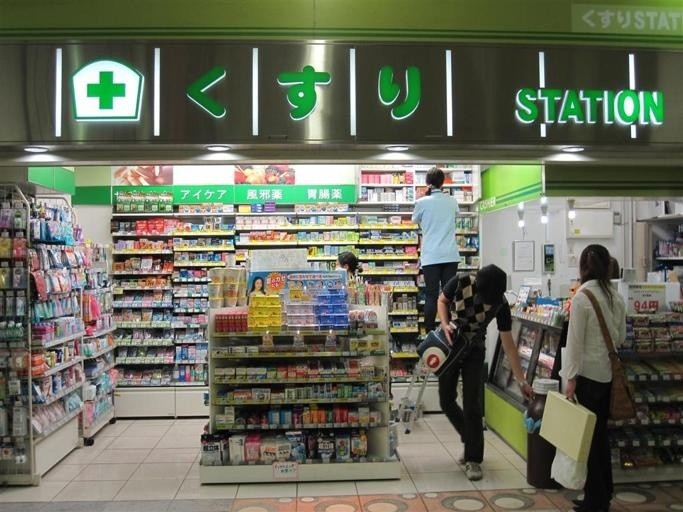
[539,391,597,464]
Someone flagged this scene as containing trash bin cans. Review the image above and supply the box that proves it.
[524,394,562,489]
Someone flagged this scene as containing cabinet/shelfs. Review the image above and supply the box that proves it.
[0,177,117,488]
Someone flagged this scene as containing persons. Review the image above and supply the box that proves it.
[410,167,459,339]
[246,276,267,304]
[559,242,628,511]
[437,265,533,479]
[335,252,362,281]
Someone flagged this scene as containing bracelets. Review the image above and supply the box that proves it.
[518,380,527,389]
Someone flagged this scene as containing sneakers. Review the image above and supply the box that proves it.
[467,461,483,481]
[571,495,613,511]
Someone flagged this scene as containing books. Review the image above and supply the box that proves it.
[493,322,558,401]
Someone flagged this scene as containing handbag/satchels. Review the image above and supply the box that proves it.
[611,362,638,420]
[417,320,469,377]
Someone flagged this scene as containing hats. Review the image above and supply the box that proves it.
[477,264,506,304]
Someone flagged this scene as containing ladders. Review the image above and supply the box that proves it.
[394,358,432,434]
